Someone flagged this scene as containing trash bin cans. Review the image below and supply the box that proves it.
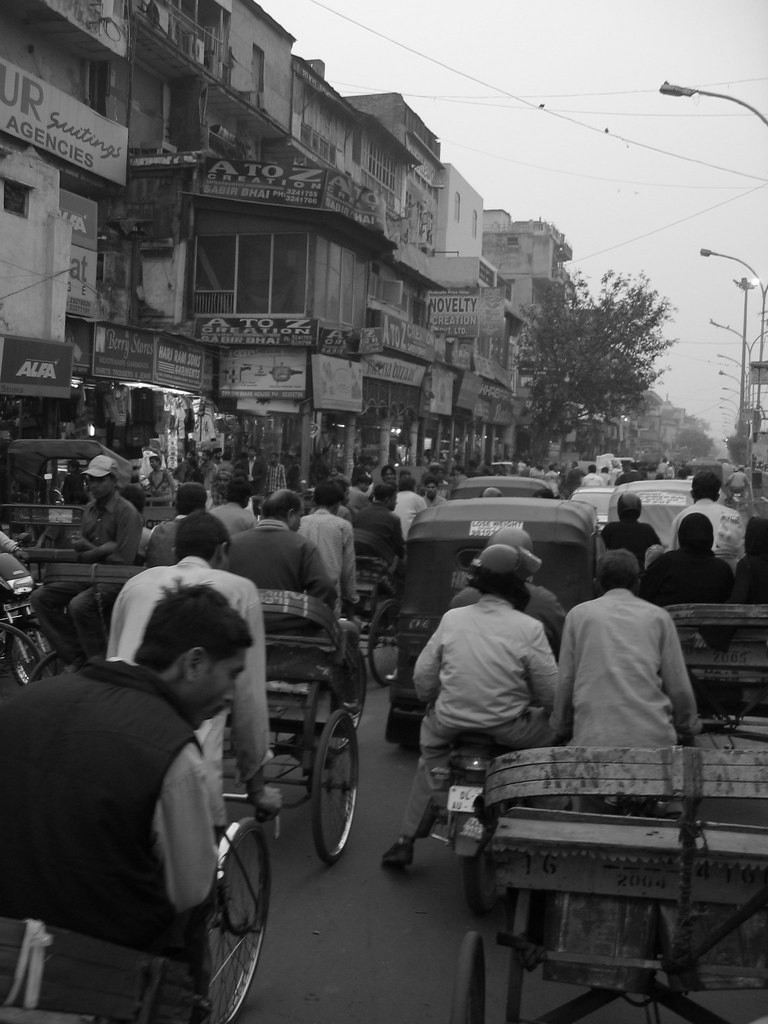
[751,470,763,490]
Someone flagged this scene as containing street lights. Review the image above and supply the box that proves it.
[709,319,768,431]
[699,248,768,472]
[733,277,761,420]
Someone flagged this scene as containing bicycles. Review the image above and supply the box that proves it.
[197,792,279,1023]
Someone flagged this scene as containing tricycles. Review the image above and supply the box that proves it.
[0,439,768,1024]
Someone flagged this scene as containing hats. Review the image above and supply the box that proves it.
[82,455,121,479]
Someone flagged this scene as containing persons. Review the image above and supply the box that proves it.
[175,449,750,594]
[412,545,558,825]
[119,484,151,555]
[548,548,702,818]
[297,480,360,621]
[104,509,281,842]
[0,531,30,563]
[601,492,660,568]
[62,460,90,505]
[145,483,208,568]
[309,475,353,522]
[0,582,253,1024]
[211,490,358,638]
[149,456,176,507]
[31,455,142,675]
[209,479,259,541]
[671,472,746,574]
[378,526,565,868]
[638,512,734,607]
[726,516,768,604]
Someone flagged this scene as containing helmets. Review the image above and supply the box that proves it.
[470,545,540,584]
[483,486,501,497]
[618,492,642,515]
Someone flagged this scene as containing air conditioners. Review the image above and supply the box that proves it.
[142,0,169,38]
[182,34,205,64]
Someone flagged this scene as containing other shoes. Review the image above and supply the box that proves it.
[381,835,411,866]
